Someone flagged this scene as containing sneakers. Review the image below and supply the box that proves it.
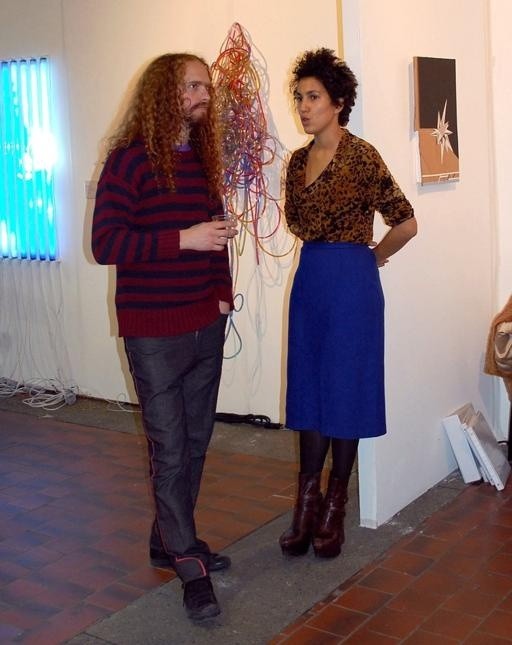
[149,547,231,623]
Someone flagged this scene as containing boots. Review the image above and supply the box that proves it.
[279,471,348,558]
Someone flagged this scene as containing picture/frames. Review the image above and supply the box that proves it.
[412,56,460,186]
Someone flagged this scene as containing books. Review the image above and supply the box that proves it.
[440,401,512,491]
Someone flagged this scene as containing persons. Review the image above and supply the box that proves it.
[89,52,241,620]
[279,46,418,560]
